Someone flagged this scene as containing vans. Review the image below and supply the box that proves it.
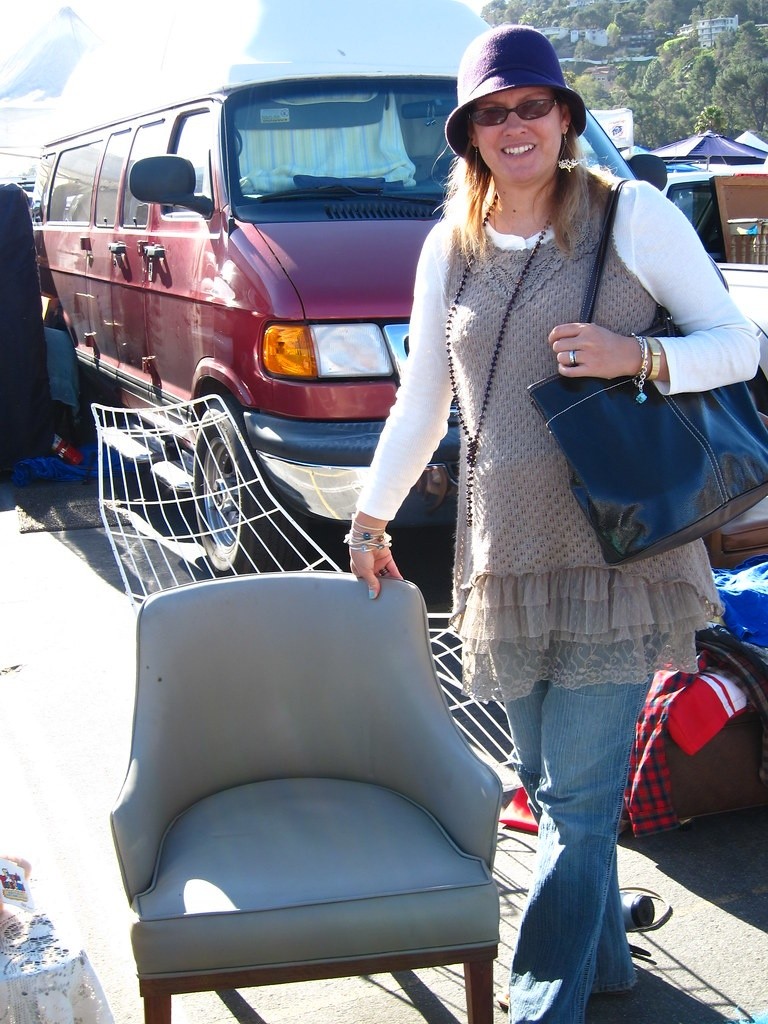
[32,71,727,576]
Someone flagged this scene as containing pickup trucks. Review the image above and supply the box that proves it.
[660,170,768,414]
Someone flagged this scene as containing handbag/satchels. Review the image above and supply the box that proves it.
[527,172,768,566]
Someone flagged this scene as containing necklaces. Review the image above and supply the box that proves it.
[445,194,555,527]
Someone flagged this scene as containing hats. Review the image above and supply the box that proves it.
[443,25,587,157]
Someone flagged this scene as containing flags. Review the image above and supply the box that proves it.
[578,110,632,152]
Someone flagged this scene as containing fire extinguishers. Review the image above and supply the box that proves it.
[51,434,84,466]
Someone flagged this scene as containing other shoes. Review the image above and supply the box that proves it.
[496,978,512,1010]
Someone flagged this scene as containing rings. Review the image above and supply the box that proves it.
[376,573,381,576]
[379,568,388,576]
[569,350,576,367]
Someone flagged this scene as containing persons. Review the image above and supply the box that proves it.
[350,25,763,1024]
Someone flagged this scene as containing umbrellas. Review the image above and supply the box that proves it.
[621,129,768,172]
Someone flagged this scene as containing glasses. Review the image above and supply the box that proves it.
[466,92,565,126]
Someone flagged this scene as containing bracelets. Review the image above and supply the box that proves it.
[343,514,392,553]
[629,333,661,404]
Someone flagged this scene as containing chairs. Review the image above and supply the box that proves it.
[109,569,503,1024]
[703,412,768,570]
